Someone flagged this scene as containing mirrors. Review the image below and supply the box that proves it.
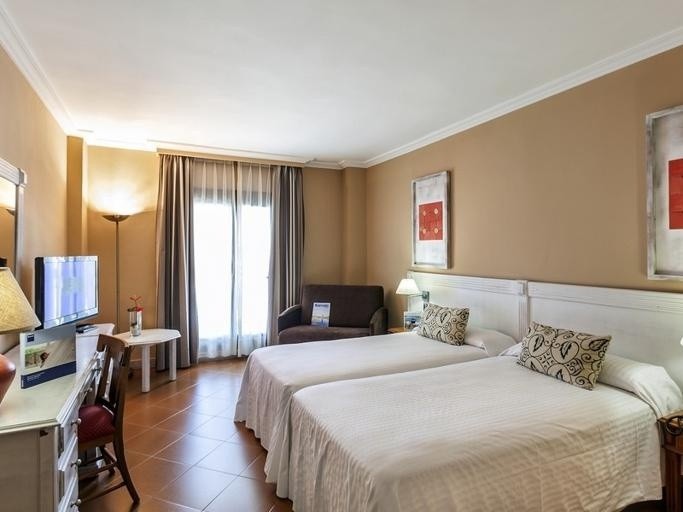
[0,156,28,285]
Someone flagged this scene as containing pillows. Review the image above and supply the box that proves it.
[517,320,612,391]
[499,339,683,448]
[412,323,517,357]
[416,301,470,346]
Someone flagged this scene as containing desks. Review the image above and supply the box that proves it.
[0,322,116,512]
[112,328,181,393]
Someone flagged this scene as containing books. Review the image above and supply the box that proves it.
[310,302,330,327]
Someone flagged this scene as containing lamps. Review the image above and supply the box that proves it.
[395,279,420,311]
[102,214,134,379]
[0,266,41,402]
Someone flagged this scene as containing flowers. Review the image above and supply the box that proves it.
[127,294,144,312]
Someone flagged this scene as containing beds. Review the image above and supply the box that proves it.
[278,281,683,511]
[234,270,529,473]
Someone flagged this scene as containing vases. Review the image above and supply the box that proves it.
[129,310,143,335]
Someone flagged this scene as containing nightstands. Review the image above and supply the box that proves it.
[656,408,683,512]
[389,327,408,334]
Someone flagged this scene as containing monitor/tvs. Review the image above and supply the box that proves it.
[34,255,99,329]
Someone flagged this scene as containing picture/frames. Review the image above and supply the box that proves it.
[643,102,683,281]
[410,171,449,270]
[404,311,422,330]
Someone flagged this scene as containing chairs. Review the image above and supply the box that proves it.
[77,333,141,504]
[277,284,389,343]
